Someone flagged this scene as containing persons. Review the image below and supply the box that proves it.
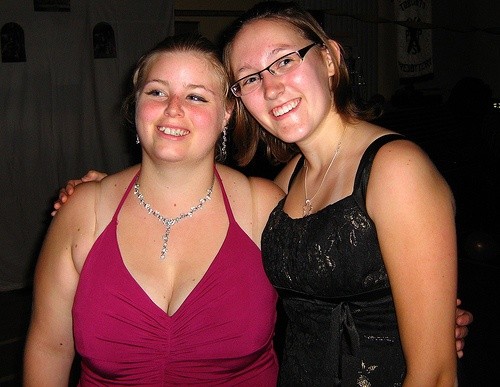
[22,35,470,387]
[52,8,458,387]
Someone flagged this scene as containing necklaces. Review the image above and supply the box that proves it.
[133,183,212,259]
[304,122,347,215]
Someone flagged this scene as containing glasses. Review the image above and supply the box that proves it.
[230,42,320,97]
